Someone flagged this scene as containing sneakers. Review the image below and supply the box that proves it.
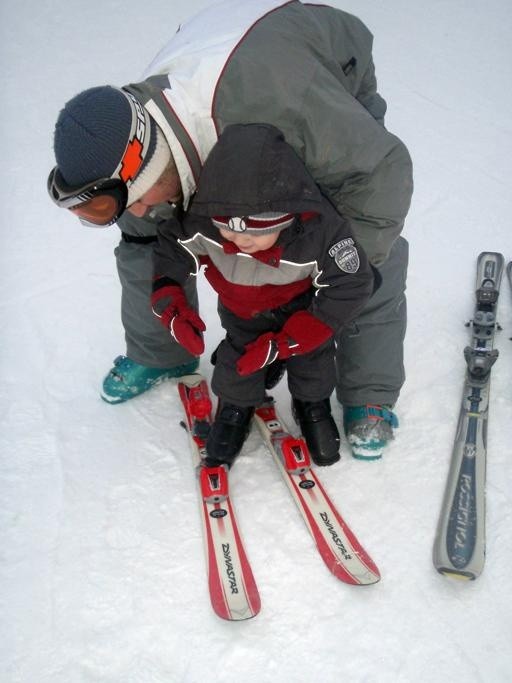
[344,401,398,460]
[205,402,255,469]
[98,353,200,404]
[292,395,343,465]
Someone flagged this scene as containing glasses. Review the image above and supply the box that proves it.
[47,142,148,228]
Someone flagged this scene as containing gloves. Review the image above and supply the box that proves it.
[236,307,336,377]
[148,274,209,361]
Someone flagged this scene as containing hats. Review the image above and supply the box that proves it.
[211,210,296,236]
[48,84,171,217]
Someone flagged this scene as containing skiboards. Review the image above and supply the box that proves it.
[431,250,511,586]
[179,366,381,623]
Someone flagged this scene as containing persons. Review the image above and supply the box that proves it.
[54,1,414,460]
[151,123,377,466]
